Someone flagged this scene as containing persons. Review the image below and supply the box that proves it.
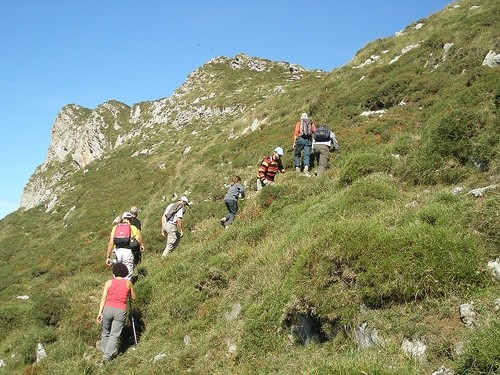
[256,147,286,191]
[105,206,144,280]
[161,196,190,257]
[292,113,339,177]
[219,176,245,229]
[96,263,137,364]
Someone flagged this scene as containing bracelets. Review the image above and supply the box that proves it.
[293,142,297,143]
[140,244,144,246]
[106,256,109,258]
